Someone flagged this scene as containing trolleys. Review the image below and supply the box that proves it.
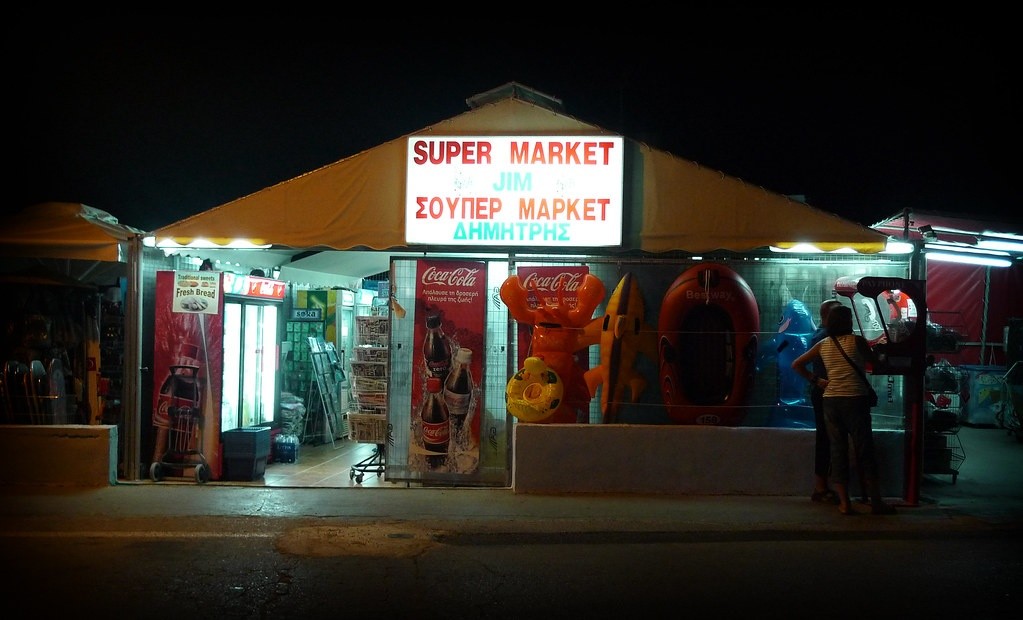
[1000,361,1023,443]
[341,412,387,484]
[149,365,210,485]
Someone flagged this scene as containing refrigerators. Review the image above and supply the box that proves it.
[151,270,287,486]
[293,287,356,440]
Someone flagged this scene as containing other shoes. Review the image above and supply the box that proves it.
[812,488,842,505]
[870,500,898,515]
[837,502,851,514]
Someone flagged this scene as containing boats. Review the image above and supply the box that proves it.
[658,262,760,425]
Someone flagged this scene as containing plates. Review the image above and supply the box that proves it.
[179,296,209,312]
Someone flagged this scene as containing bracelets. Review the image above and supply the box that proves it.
[811,375,817,384]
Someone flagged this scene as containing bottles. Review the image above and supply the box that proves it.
[152,343,199,474]
[421,378,450,468]
[422,314,453,382]
[443,347,473,441]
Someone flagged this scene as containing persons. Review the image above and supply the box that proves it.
[30,316,70,369]
[808,300,842,505]
[791,305,898,514]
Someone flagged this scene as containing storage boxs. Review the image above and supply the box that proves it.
[221,426,271,481]
[272,443,299,463]
[959,365,1007,428]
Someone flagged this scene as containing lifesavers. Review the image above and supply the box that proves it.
[854,290,918,374]
[505,358,564,422]
[658,262,759,424]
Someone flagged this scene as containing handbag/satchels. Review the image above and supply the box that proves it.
[866,385,878,406]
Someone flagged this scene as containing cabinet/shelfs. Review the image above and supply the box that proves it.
[923,310,970,476]
